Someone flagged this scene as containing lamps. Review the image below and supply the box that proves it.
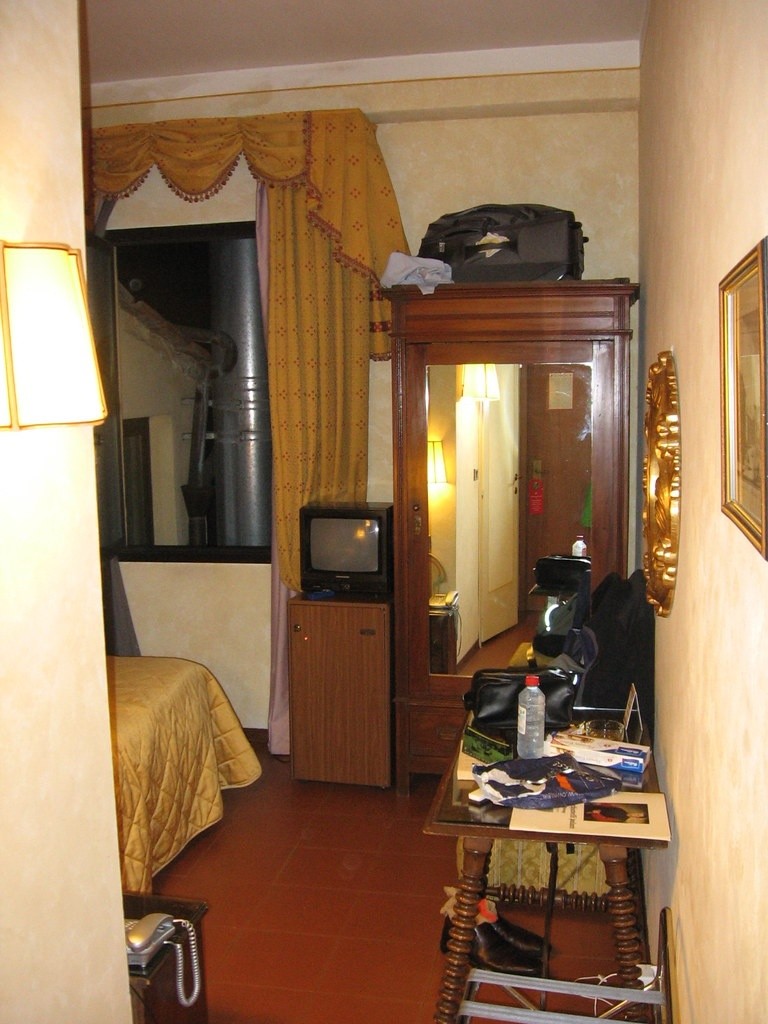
[0,241,109,432]
[426,440,448,486]
[461,365,502,403]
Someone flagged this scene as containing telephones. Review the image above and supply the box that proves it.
[124,913,178,969]
[429,591,460,608]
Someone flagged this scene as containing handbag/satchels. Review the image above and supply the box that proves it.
[534,555,592,590]
[461,665,574,731]
[532,592,579,658]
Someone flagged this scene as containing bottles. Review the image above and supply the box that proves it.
[516,675,546,761]
[572,535,587,556]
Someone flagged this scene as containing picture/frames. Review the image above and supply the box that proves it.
[719,235,768,562]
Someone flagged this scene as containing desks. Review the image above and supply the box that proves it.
[421,708,671,1024]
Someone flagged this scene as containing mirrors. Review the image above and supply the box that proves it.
[423,363,593,676]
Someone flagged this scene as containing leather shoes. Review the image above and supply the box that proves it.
[486,916,551,956]
[438,911,548,978]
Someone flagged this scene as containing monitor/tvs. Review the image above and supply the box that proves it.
[300,500,393,594]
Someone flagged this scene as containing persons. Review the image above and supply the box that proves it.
[584,804,647,822]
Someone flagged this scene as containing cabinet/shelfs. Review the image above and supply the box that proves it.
[425,363,521,662]
[287,593,393,788]
[378,275,640,797]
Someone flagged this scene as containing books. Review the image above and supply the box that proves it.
[510,793,672,842]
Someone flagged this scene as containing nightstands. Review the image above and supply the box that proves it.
[122,891,208,1024]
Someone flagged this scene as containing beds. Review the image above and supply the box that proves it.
[104,654,263,895]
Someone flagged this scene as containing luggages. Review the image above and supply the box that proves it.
[418,204,589,282]
